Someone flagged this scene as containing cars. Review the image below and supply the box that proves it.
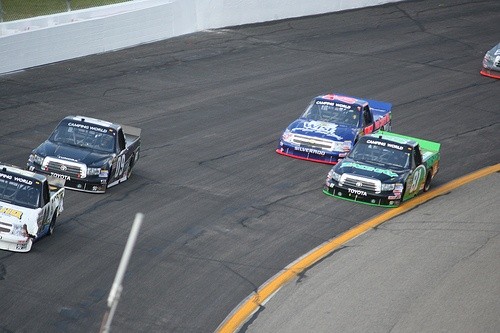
[26,117,142,193]
[323,129,441,207]
[0,162,65,253]
[276,93,392,164]
[480,42,500,78]
